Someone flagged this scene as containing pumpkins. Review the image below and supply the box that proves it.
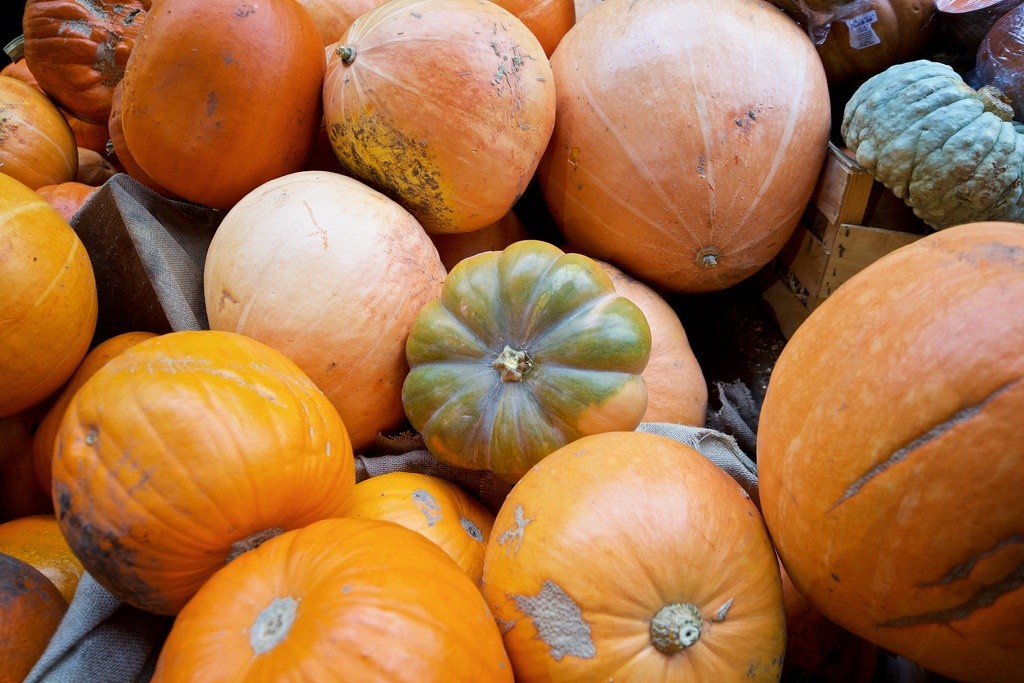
[3,0,1024,683]
